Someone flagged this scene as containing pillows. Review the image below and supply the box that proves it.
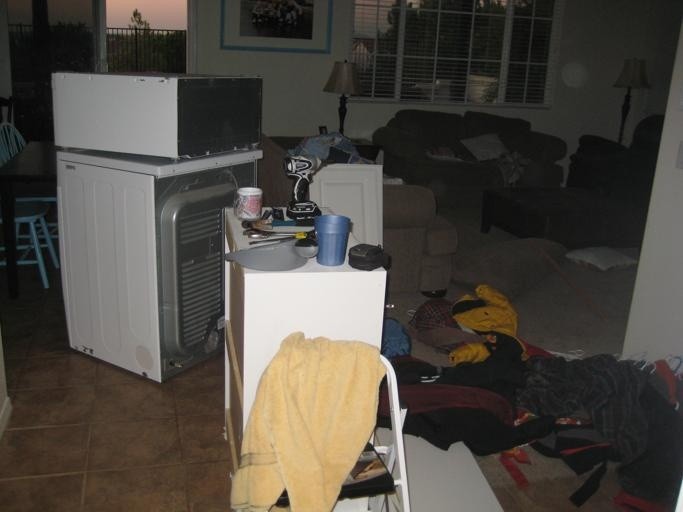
[565,243,638,272]
[573,135,630,179]
[453,237,569,300]
[395,109,532,167]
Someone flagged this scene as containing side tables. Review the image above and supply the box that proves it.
[345,137,382,160]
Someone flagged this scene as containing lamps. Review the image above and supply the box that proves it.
[322,59,362,139]
[612,57,651,143]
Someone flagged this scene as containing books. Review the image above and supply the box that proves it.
[271,218,315,234]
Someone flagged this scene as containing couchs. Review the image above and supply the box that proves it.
[373,117,567,224]
[256,131,458,297]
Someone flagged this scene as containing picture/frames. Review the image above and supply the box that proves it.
[220,0,333,53]
[318,125,328,135]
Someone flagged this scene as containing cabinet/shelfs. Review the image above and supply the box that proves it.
[220,208,388,477]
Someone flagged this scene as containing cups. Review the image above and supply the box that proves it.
[314,214,351,266]
[236,187,264,221]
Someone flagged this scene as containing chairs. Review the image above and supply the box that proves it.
[0,96,60,299]
[565,114,664,215]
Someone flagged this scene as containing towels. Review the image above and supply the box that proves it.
[229,330,387,512]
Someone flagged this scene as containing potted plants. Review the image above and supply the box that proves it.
[463,36,504,104]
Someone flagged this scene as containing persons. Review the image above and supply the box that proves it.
[250,1,269,24]
[62,21,95,71]
[268,1,281,22]
[282,1,303,25]
